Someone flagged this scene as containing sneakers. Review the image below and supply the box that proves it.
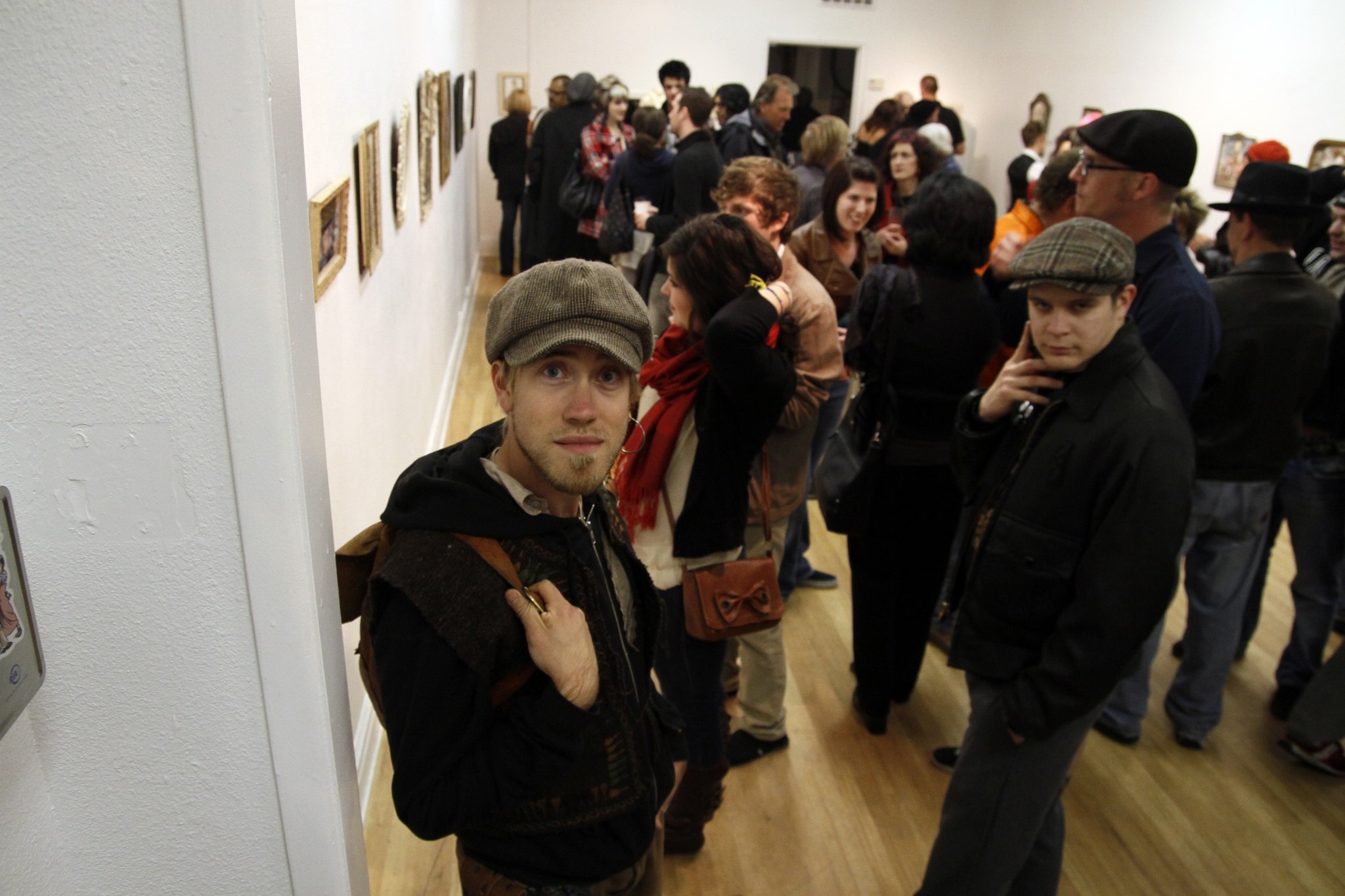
[1282,732,1344,777]
[1174,727,1202,751]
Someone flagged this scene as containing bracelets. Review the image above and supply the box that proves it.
[774,282,793,314]
[767,286,784,321]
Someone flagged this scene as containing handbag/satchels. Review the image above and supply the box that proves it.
[559,127,605,221]
[682,556,782,642]
[598,161,636,255]
[814,392,892,533]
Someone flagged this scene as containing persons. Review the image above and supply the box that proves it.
[929,110,1222,784]
[715,74,800,167]
[633,87,727,349]
[1169,140,1345,777]
[913,216,1195,896]
[777,76,965,601]
[603,106,676,288]
[354,258,689,896]
[602,214,798,856]
[659,61,690,155]
[578,75,636,262]
[844,169,1003,735]
[976,120,1082,390]
[1090,161,1340,749]
[525,73,598,271]
[710,156,844,767]
[488,89,532,277]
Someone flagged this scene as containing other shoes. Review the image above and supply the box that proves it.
[1271,690,1296,721]
[800,571,836,587]
[1171,639,1246,662]
[726,730,789,765]
[1090,723,1139,745]
[931,747,963,773]
[659,810,705,855]
[928,624,955,655]
[849,660,904,705]
[852,687,886,736]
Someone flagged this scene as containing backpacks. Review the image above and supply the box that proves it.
[334,518,546,734]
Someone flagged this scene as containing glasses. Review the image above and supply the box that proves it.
[1078,150,1150,179]
[547,86,564,96]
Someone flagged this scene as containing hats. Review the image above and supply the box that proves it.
[485,259,653,376]
[1076,109,1198,189]
[1208,161,1331,213]
[1010,217,1136,295]
